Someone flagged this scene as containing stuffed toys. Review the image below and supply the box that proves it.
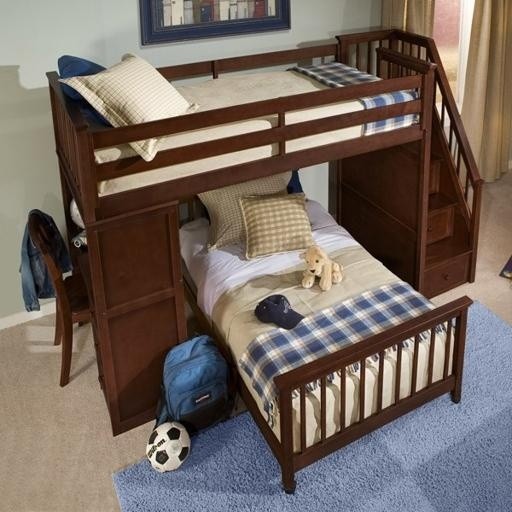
[298,246,343,292]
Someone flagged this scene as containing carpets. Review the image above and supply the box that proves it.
[111,301,511,510]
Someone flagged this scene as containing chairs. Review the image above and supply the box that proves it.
[27,211,94,388]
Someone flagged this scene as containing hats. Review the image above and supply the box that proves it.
[253,293,305,331]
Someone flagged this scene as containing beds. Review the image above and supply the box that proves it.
[46,28,483,437]
[175,168,472,494]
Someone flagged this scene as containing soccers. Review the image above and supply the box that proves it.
[145,422,190,472]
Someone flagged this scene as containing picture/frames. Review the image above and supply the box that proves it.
[139,1,292,47]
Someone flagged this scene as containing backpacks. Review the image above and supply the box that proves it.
[149,333,239,436]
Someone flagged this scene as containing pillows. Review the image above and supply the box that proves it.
[195,171,314,260]
[58,54,198,163]
